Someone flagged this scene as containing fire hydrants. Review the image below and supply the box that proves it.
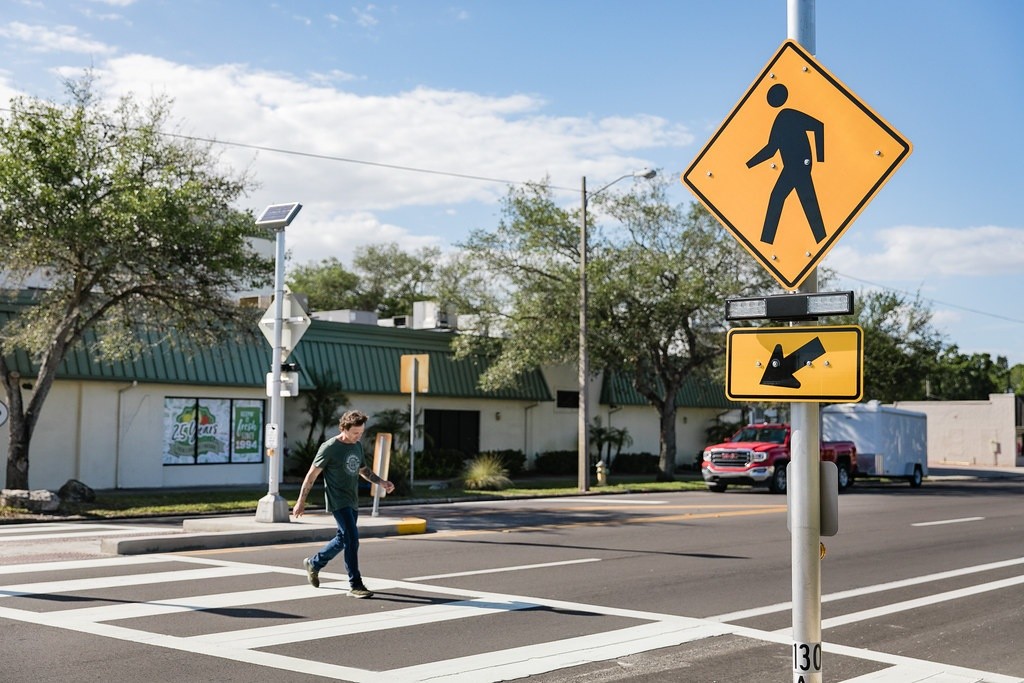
[596,459,610,485]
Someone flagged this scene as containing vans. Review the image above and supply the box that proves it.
[820,400,931,486]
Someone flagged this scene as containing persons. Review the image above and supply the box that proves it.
[293,410,395,598]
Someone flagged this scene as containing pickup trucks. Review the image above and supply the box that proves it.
[700,421,858,494]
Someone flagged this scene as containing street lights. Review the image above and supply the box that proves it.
[253,202,306,524]
[574,169,660,491]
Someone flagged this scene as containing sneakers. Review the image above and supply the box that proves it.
[346,584,373,598]
[303,558,319,588]
